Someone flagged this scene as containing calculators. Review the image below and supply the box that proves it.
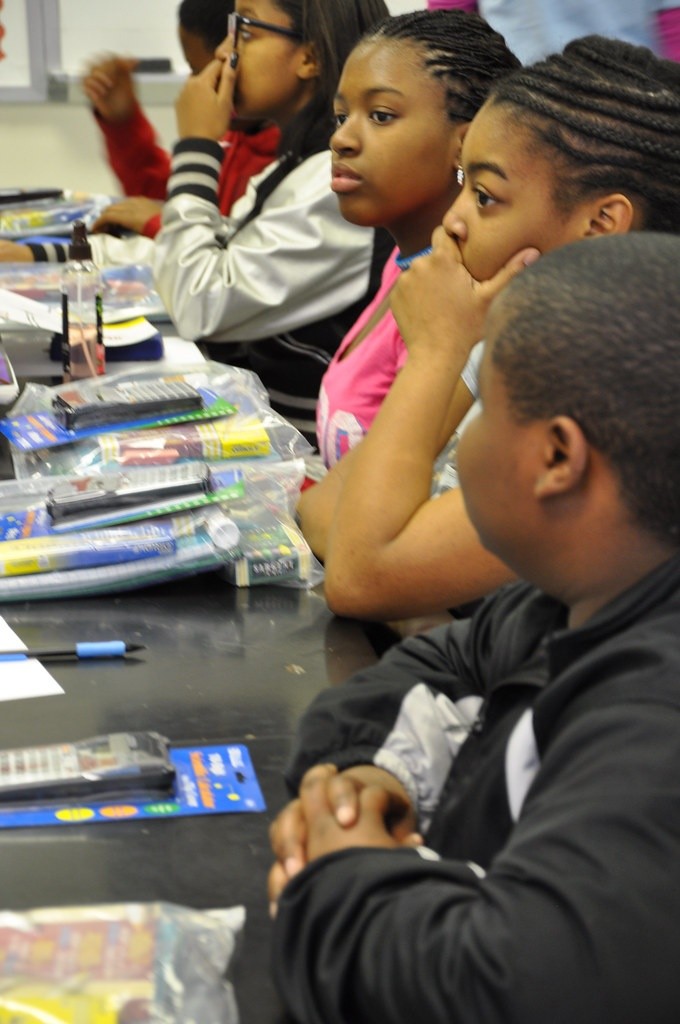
[51,380,203,430]
[45,460,209,521]
[0,730,176,812]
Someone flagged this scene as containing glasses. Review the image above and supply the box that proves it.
[225,11,300,49]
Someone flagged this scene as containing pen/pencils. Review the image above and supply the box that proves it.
[0,640,147,674]
[61,293,71,372]
[95,291,107,375]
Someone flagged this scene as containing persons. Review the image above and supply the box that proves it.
[162,0,394,458]
[425,0,680,64]
[269,228,680,1023]
[287,30,679,645]
[318,9,524,480]
[77,0,287,241]
